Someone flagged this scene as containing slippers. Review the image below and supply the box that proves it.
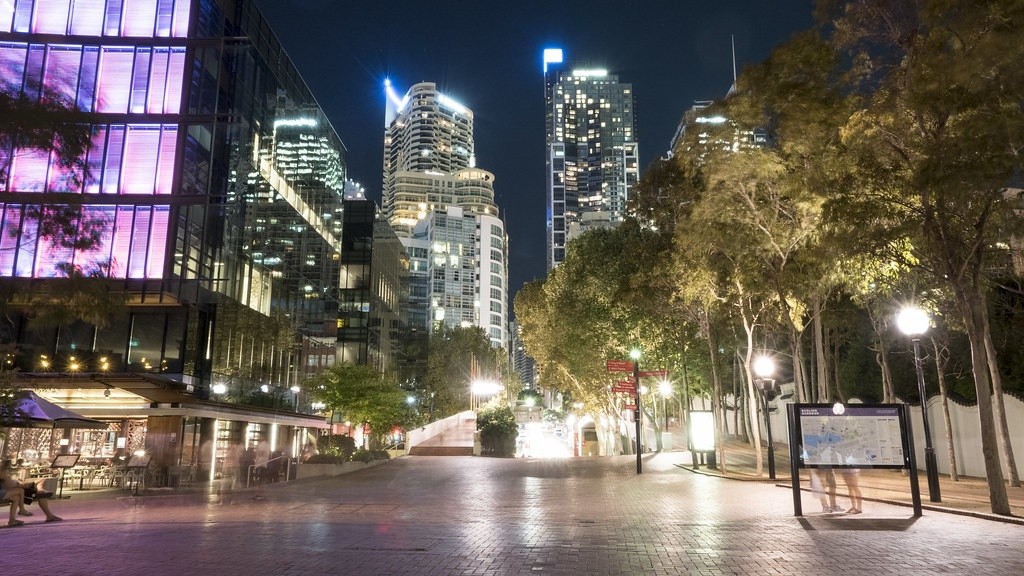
[45,516,63,522]
[35,492,52,499]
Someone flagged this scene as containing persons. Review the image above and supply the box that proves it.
[37,452,46,465]
[839,469,862,513]
[0,460,33,525]
[810,467,845,512]
[124,453,130,462]
[18,477,62,522]
[112,453,120,462]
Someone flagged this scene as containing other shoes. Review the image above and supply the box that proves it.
[852,509,862,514]
[823,505,833,513]
[18,512,33,516]
[8,520,24,525]
[847,508,855,513]
[830,505,845,512]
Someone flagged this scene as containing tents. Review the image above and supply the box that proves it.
[0,390,108,430]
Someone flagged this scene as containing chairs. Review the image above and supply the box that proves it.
[20,464,197,491]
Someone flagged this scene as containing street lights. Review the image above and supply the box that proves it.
[753,353,780,479]
[896,304,944,503]
[629,347,645,474]
